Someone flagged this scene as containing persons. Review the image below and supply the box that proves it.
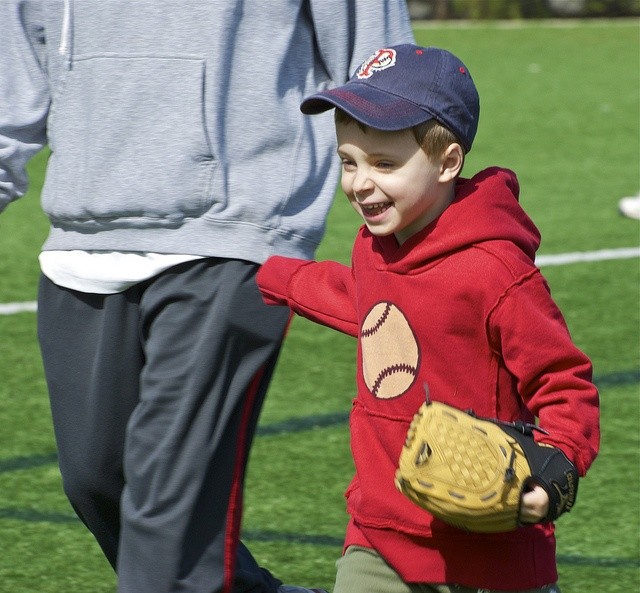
[0,0,415,469]
[255,43,598,593]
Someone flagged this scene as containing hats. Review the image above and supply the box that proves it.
[300,44,480,155]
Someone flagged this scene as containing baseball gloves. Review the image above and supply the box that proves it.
[394,381,578,533]
